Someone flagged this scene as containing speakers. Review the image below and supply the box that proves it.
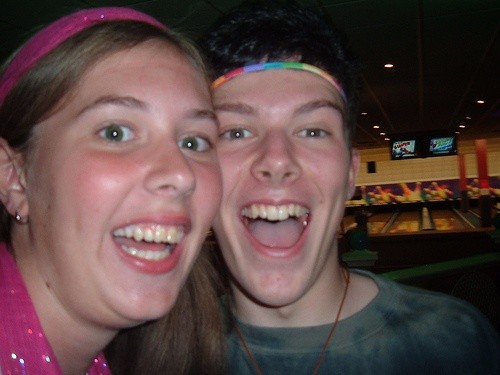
[367,161,375,173]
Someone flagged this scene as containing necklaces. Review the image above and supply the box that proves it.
[226,263,350,375]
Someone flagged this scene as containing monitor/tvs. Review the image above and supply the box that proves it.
[429,137,455,157]
[391,139,416,160]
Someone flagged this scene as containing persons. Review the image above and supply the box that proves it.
[0,8,221,375]
[206,9,500,375]
